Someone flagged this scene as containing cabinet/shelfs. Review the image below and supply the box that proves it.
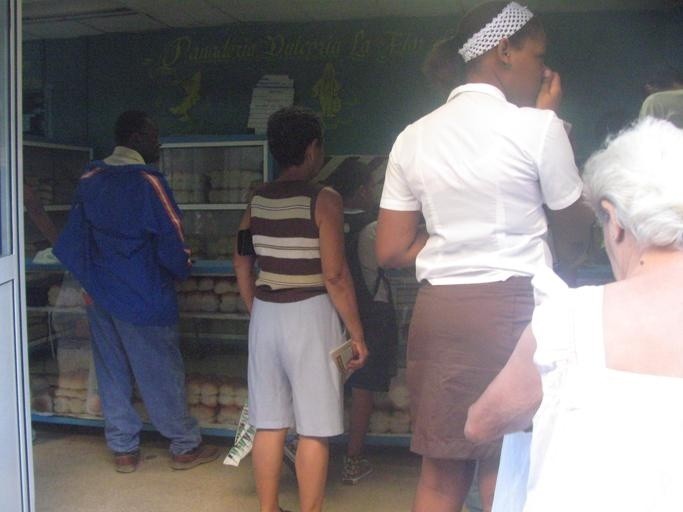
[20,136,419,447]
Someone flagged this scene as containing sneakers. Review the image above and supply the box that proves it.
[284,431,298,472]
[339,449,374,484]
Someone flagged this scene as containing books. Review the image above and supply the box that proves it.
[327,338,360,387]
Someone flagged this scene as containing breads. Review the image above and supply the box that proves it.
[31,373,248,434]
[30,169,262,314]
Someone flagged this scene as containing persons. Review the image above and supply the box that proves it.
[228,106,371,512]
[373,2,589,512]
[462,115,682,512]
[635,52,682,130]
[280,158,401,486]
[50,107,224,476]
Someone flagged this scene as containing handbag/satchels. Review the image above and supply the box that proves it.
[235,228,256,258]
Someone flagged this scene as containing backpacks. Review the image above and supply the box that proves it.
[338,211,380,343]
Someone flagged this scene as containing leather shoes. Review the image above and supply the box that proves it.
[111,449,140,473]
[171,441,220,470]
